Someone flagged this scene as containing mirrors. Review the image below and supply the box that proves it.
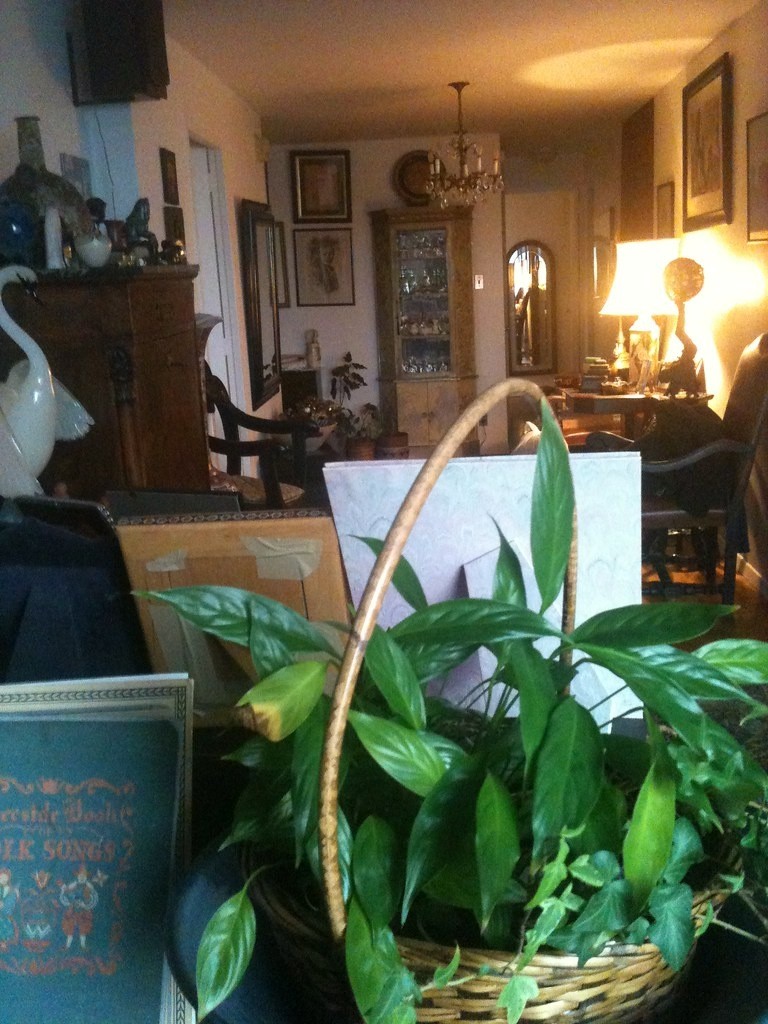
[505,240,558,377]
[234,201,280,412]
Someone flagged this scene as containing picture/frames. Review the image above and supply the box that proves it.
[159,147,181,206]
[164,206,186,248]
[295,228,356,306]
[680,52,736,230]
[275,222,288,309]
[289,147,353,222]
[391,150,447,206]
[654,181,676,239]
[744,112,768,243]
[589,205,615,300]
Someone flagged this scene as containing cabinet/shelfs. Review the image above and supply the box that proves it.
[2,268,210,506]
[375,209,478,453]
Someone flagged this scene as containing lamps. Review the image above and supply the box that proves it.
[422,82,505,206]
[599,242,702,375]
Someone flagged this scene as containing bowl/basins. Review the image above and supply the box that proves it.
[601,383,632,394]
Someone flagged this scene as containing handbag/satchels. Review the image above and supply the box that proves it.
[623,402,754,519]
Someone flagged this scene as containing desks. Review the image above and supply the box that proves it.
[281,367,326,408]
[562,387,709,439]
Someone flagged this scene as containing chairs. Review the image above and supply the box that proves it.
[204,360,320,506]
[584,333,768,621]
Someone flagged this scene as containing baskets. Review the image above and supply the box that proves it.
[242,377,743,1024]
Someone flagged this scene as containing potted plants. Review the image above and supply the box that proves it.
[160,383,768,1023]
[324,350,410,458]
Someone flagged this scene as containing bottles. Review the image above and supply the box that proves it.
[400,233,444,258]
[401,312,449,335]
[618,357,629,382]
[401,267,447,293]
[403,355,448,373]
